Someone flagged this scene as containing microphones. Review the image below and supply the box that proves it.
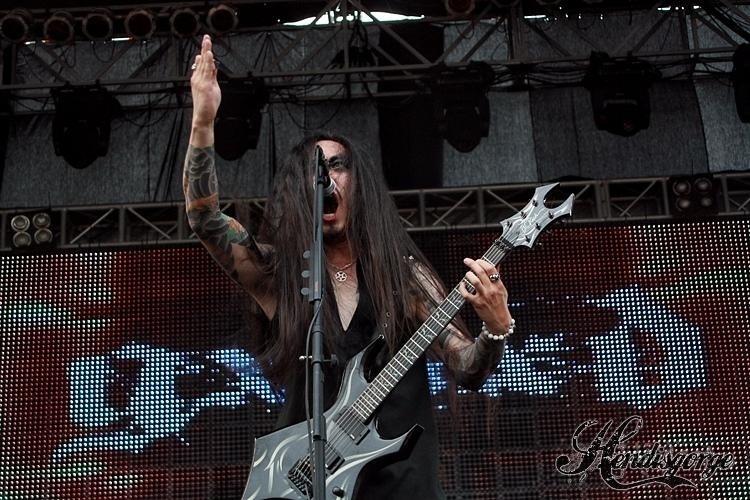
[311,145,337,196]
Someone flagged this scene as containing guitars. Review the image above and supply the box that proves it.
[241,182,575,500]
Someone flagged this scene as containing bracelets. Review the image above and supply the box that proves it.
[481,318,516,341]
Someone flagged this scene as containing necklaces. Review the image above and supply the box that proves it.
[325,257,357,282]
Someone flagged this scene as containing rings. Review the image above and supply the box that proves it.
[189,63,197,72]
[489,273,500,282]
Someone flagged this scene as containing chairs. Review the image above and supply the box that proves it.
[0,0,750,169]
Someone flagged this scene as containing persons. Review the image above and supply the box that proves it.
[182,33,518,500]
